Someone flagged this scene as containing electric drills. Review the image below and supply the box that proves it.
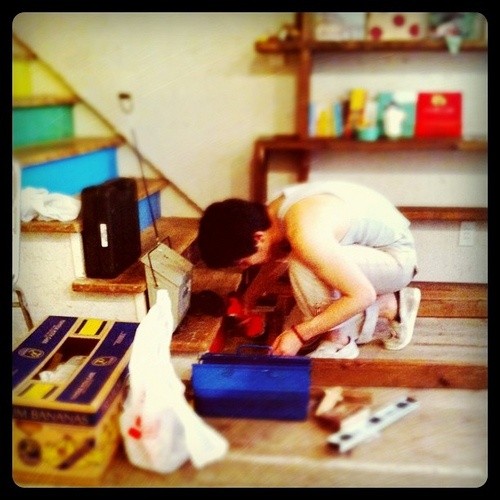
[221,292,270,362]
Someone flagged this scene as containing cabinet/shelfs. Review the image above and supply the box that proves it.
[250,10,488,319]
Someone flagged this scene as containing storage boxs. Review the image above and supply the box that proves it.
[12,316,142,488]
[191,343,313,420]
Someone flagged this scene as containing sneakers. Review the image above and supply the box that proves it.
[303,335,361,360]
[380,285,421,350]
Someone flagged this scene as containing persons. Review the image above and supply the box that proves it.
[197,180,421,361]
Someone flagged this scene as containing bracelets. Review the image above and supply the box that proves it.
[289,323,307,345]
